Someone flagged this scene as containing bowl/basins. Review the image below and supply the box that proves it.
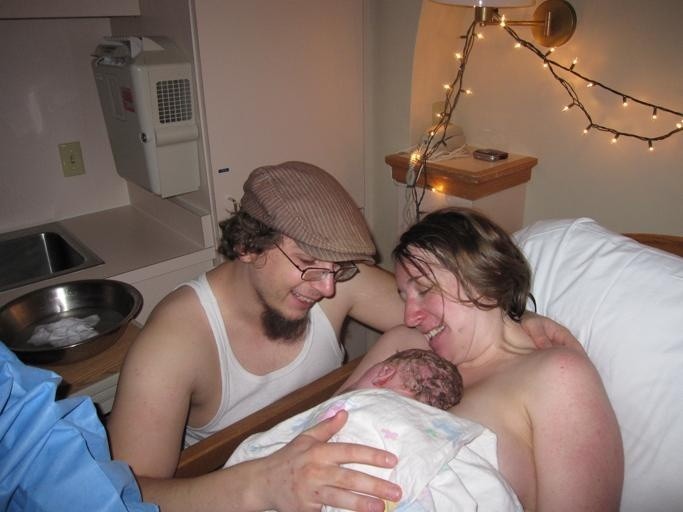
[0,280,144,366]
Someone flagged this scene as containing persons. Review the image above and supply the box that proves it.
[331,206,624,512]
[353,348,464,411]
[107,162,587,512]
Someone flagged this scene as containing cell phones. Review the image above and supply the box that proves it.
[473,148,508,161]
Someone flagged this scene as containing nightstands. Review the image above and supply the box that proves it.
[384,143,538,238]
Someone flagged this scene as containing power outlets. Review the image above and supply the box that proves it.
[57,142,85,179]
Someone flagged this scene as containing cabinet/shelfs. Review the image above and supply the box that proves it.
[64,207,213,322]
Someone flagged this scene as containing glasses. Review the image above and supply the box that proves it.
[274,243,360,283]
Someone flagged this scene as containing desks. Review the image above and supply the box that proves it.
[32,322,145,419]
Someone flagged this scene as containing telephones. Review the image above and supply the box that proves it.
[418,123,466,158]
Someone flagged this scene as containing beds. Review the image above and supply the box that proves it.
[142,219,682,512]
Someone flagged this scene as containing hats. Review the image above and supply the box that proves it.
[240,161,376,266]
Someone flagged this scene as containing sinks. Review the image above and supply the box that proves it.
[0,221,106,292]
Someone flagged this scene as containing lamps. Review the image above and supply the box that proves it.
[433,0,577,47]
